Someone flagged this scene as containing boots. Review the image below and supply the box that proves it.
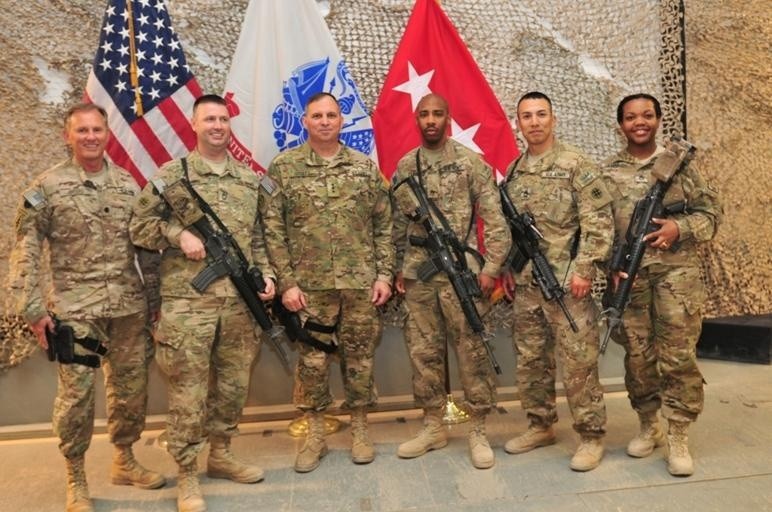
[176,455,206,511]
[64,453,93,512]
[108,444,166,490]
[293,407,330,473]
[624,409,667,457]
[468,409,494,469]
[348,405,375,463]
[665,419,694,476]
[206,434,264,483]
[397,407,448,458]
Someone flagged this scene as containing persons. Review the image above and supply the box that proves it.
[390,92,515,471]
[503,90,617,473]
[8,101,163,512]
[597,92,722,478]
[258,92,396,475]
[128,94,278,512]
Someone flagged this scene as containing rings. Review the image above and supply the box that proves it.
[661,240,670,250]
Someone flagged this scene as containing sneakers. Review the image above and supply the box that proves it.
[570,435,606,471]
[504,423,556,453]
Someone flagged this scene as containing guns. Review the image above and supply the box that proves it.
[599,133,697,355]
[496,183,580,333]
[391,173,503,375]
[160,178,294,394]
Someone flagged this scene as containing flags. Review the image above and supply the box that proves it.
[372,0,522,304]
[221,1,379,189]
[80,0,204,190]
